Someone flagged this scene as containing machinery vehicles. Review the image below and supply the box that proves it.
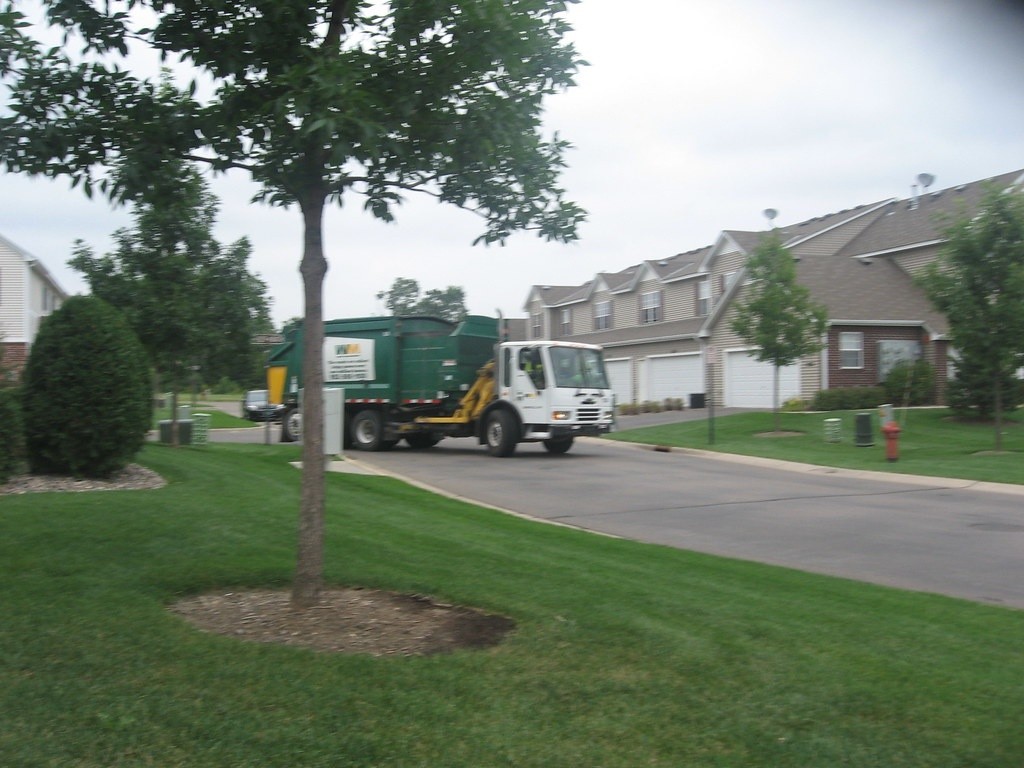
[267,314,616,457]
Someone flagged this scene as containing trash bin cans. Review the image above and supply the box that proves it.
[157,418,194,446]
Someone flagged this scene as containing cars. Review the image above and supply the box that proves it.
[241,388,286,422]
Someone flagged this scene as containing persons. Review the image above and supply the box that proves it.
[525,351,544,388]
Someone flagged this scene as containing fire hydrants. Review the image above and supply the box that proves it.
[880,420,901,462]
